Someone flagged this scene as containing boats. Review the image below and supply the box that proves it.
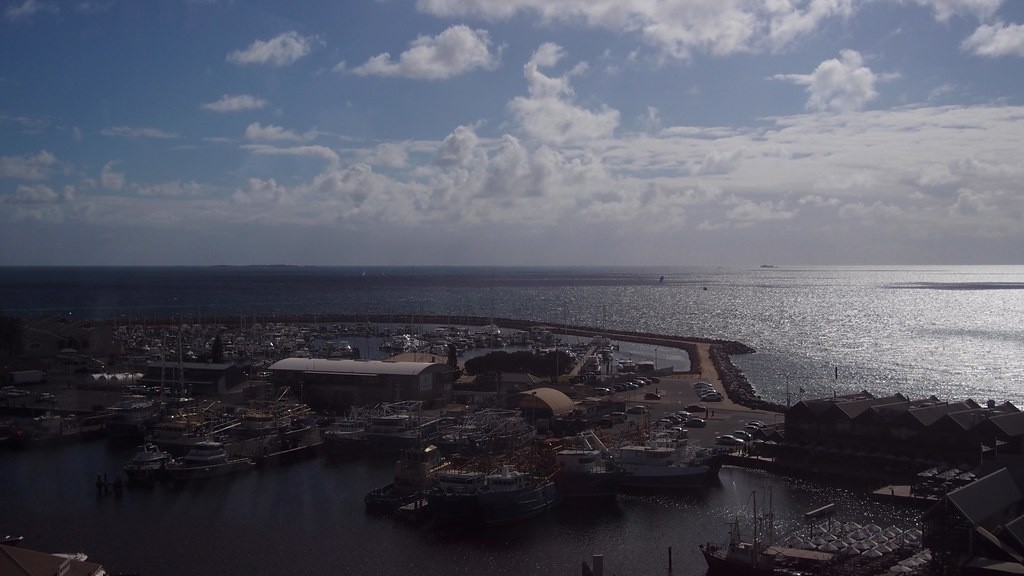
[1,317,776,527]
